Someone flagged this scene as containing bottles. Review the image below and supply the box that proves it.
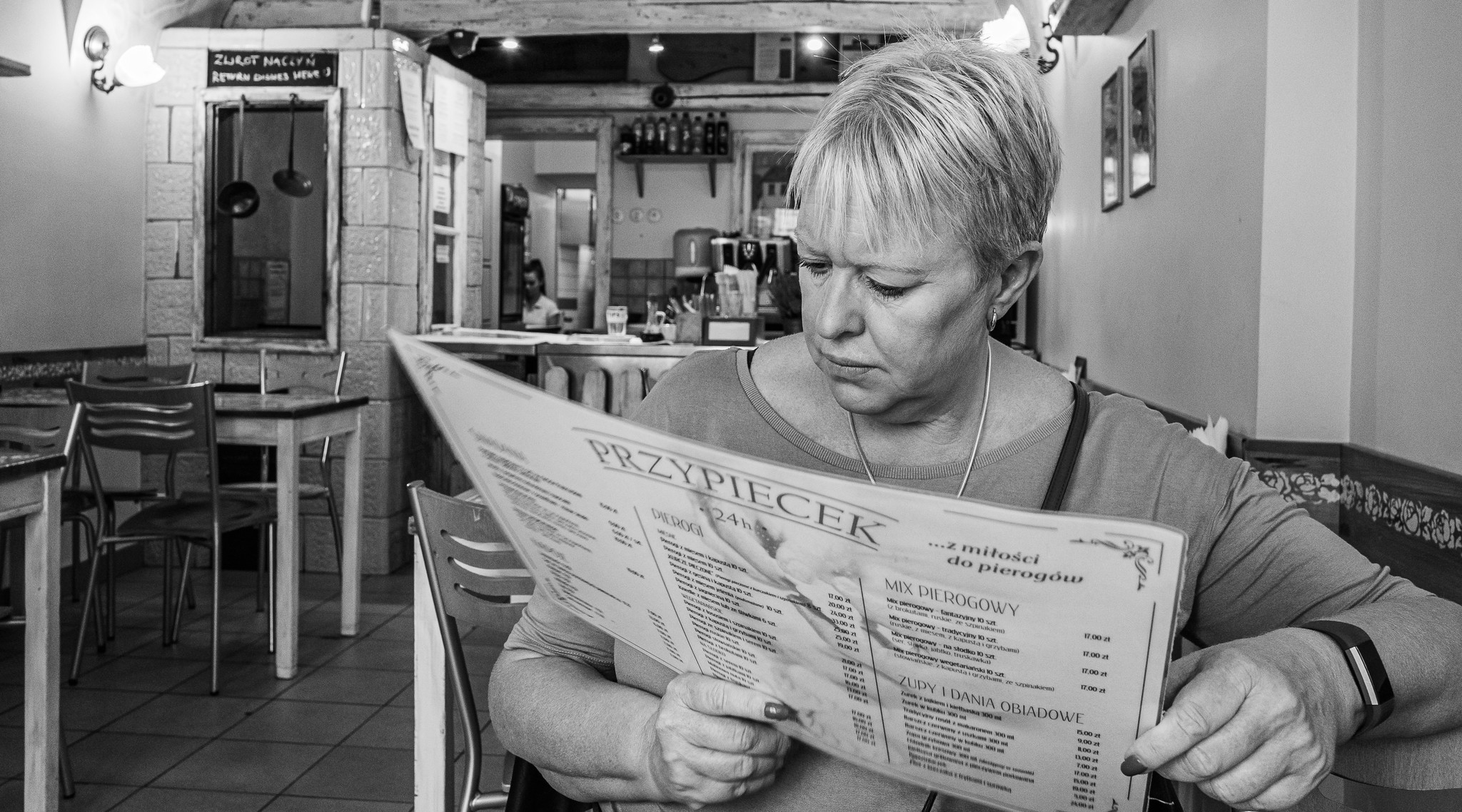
[620,111,729,157]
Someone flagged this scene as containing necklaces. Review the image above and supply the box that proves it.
[840,335,994,499]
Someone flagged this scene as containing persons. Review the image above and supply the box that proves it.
[484,29,1462,812]
[521,258,560,332]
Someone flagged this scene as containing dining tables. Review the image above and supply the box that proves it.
[0,451,61,812]
[400,482,538,812]
[0,385,372,683]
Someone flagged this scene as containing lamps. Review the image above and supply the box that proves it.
[503,38,518,49]
[648,34,664,54]
[980,4,1063,75]
[84,26,167,94]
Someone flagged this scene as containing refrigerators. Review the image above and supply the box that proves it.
[502,183,528,322]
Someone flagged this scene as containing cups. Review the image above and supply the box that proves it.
[693,273,757,317]
[606,305,629,337]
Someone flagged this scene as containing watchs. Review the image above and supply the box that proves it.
[1294,619,1398,740]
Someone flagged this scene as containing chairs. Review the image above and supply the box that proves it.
[51,360,197,638]
[0,404,102,677]
[408,476,540,812]
[171,345,343,642]
[64,377,281,696]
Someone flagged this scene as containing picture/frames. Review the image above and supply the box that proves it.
[1127,28,1156,199]
[1099,66,1123,212]
